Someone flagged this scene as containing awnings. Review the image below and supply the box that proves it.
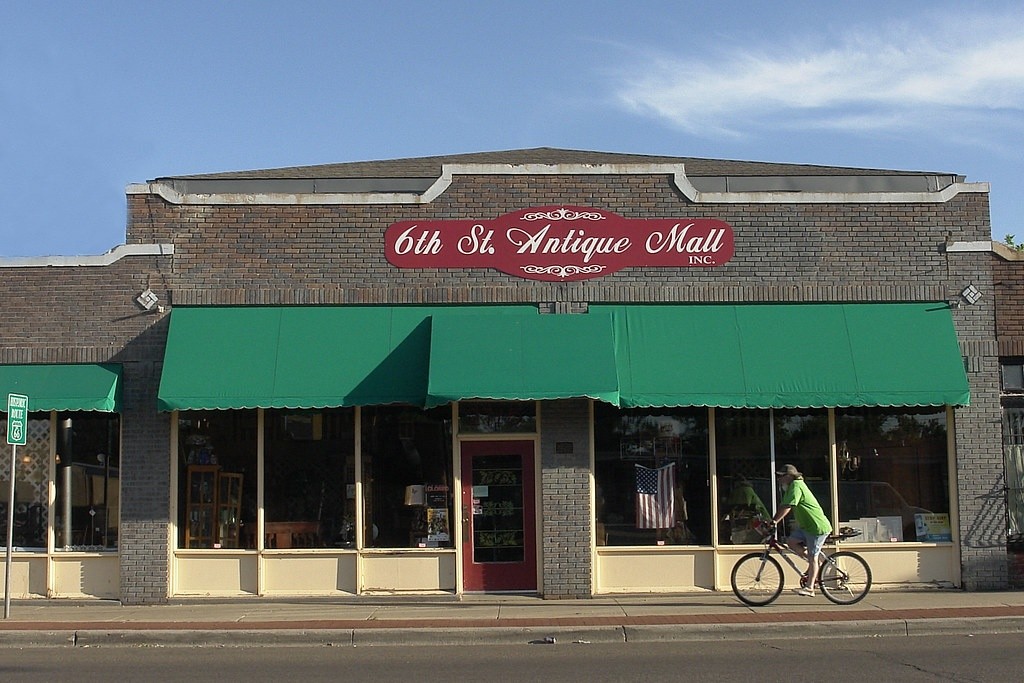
[154,299,970,415]
[0,363,124,415]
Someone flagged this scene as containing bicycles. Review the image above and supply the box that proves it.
[730,517,873,606]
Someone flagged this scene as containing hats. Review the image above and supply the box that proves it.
[730,474,750,486]
[776,464,802,475]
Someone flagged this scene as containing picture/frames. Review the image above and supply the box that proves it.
[399,421,415,439]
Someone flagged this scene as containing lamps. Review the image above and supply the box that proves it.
[824,439,862,474]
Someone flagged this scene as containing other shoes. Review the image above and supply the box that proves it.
[793,588,815,597]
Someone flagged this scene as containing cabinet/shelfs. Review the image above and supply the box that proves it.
[185,465,243,549]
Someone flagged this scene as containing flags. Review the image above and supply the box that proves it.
[635,461,674,528]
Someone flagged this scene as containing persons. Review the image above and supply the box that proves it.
[728,473,774,531]
[765,464,833,597]
